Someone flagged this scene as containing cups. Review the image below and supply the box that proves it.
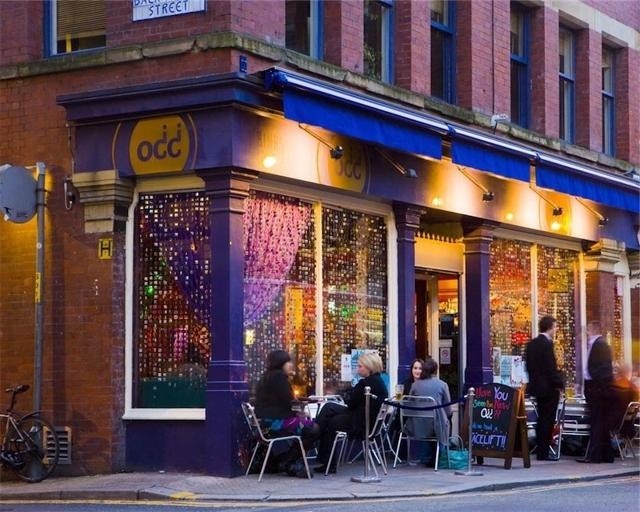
[565,387,577,403]
[395,385,405,400]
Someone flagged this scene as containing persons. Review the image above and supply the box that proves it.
[403,359,452,442]
[257,350,320,478]
[527,316,565,461]
[178,343,205,377]
[612,360,640,433]
[314,352,388,473]
[576,320,614,463]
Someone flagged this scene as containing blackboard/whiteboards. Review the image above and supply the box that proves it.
[460,383,521,458]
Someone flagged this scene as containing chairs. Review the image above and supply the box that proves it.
[525,399,639,459]
[242,396,452,481]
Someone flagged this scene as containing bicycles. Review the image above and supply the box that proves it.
[0,383,60,482]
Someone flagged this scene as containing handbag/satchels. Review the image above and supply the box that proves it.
[439,435,469,470]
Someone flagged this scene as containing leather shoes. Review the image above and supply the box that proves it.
[576,455,614,463]
[536,452,560,461]
[313,465,336,474]
